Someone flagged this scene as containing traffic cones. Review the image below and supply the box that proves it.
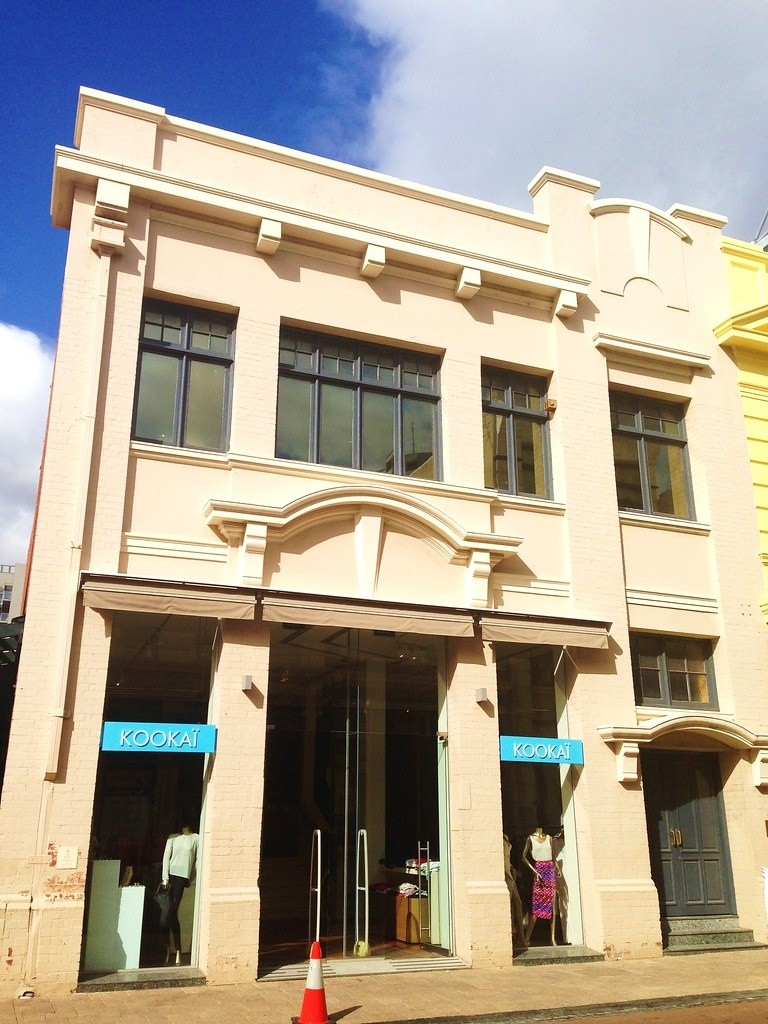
[290,941,337,1024]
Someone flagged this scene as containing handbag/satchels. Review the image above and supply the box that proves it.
[149,884,171,925]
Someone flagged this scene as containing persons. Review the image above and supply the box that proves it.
[521,826,562,947]
[161,826,200,966]
[502,833,529,948]
